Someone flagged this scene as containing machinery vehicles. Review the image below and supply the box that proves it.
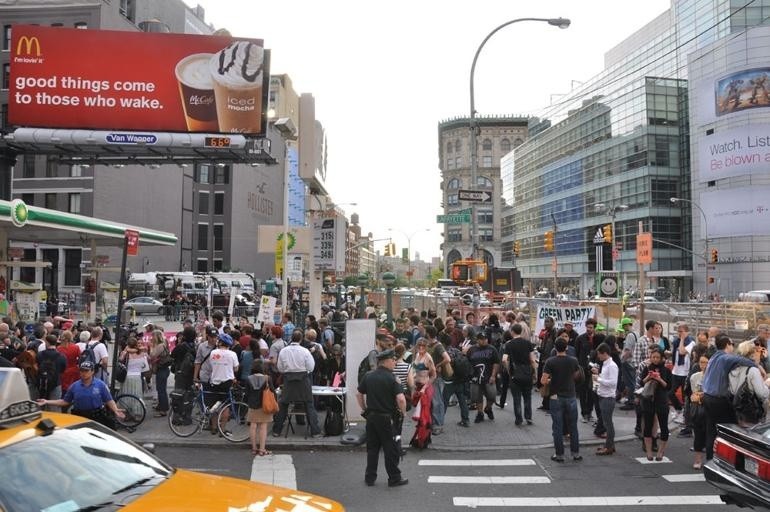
[127,271,275,305]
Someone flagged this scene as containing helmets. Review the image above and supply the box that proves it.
[594,317,636,333]
[217,333,234,346]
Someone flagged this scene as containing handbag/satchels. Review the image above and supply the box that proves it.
[114,360,127,383]
[263,387,280,415]
[731,378,765,426]
[159,345,175,369]
[412,399,421,421]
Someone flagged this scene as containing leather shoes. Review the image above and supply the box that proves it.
[595,447,613,456]
[656,453,664,461]
[364,480,374,486]
[388,478,408,487]
[693,462,703,470]
[597,446,616,453]
[647,454,653,461]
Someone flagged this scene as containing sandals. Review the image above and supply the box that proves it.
[258,449,273,456]
[251,449,259,455]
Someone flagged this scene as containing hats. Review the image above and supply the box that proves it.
[143,321,153,327]
[207,327,219,337]
[375,347,396,361]
[79,361,95,370]
[477,330,490,339]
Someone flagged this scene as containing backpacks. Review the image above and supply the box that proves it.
[331,325,346,348]
[174,351,196,379]
[452,351,475,385]
[35,351,62,396]
[78,341,101,376]
[322,407,344,438]
[15,348,40,382]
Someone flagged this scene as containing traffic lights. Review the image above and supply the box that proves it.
[452,263,469,281]
[709,246,719,265]
[436,214,471,224]
[447,208,472,214]
[710,277,714,283]
[514,241,519,254]
[385,244,390,257]
[602,224,611,243]
[458,189,492,203]
[543,232,553,251]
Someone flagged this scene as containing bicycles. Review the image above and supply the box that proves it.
[169,378,254,443]
[102,383,146,429]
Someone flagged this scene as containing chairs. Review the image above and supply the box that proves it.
[285,399,311,439]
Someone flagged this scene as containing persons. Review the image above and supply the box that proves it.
[540,336,584,463]
[590,341,618,456]
[637,346,668,461]
[0,277,770,441]
[689,352,713,470]
[355,347,409,487]
[245,362,274,455]
[701,334,752,459]
[632,343,661,451]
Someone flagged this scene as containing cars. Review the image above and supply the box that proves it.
[1,367,347,512]
[123,297,168,316]
[702,422,769,512]
[228,293,257,316]
[40,298,71,313]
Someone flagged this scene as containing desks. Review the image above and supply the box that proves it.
[275,386,350,432]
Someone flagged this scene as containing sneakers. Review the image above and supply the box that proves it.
[551,454,565,462]
[572,455,583,461]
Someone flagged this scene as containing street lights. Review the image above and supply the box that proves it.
[594,203,628,271]
[358,274,368,318]
[467,14,572,324]
[387,225,430,290]
[141,255,149,273]
[334,275,344,311]
[308,191,359,219]
[383,271,398,332]
[670,195,709,303]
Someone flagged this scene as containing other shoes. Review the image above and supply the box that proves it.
[247,423,251,426]
[582,415,589,423]
[212,428,218,435]
[172,418,192,425]
[672,408,685,420]
[475,412,484,423]
[458,418,469,427]
[154,412,167,418]
[537,406,550,411]
[526,419,533,425]
[484,407,494,420]
[680,428,689,435]
[432,427,443,435]
[272,429,281,437]
[239,418,246,425]
[219,430,232,438]
[589,416,597,422]
[496,402,505,408]
[674,419,687,425]
[449,401,458,407]
[624,401,635,406]
[296,419,306,426]
[515,420,523,425]
[634,430,643,438]
[152,405,159,410]
[620,405,634,410]
[312,433,322,439]
[469,405,477,410]
